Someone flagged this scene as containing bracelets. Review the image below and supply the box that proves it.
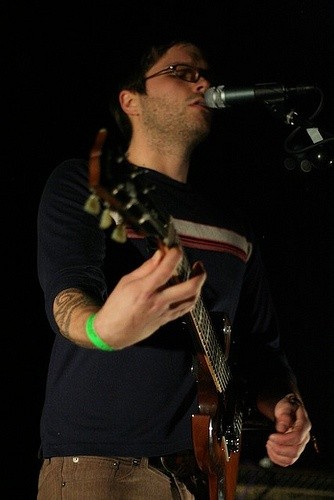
[85,313,120,351]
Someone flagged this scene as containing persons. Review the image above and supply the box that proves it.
[38,38,312,500]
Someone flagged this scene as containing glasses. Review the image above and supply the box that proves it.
[145,64,210,84]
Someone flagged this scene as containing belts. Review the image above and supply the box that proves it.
[149,454,210,495]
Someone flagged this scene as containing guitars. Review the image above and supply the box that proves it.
[84,129,247,499]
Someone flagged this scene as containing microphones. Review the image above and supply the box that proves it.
[204,80,319,108]
